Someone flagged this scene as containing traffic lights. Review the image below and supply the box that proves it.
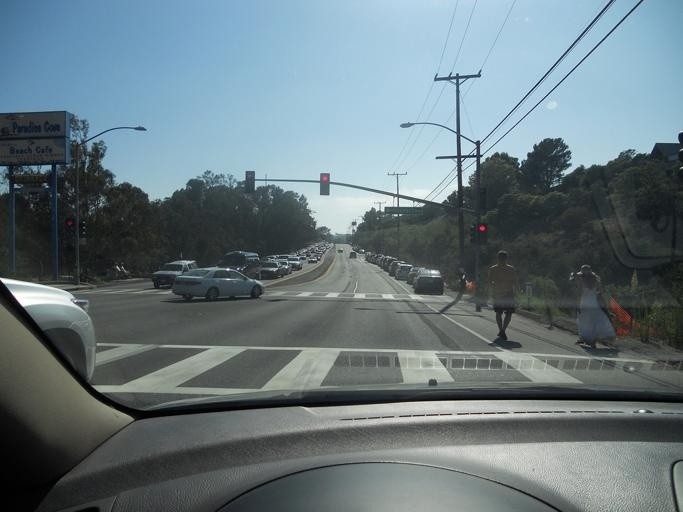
[320,172,330,196]
[476,224,488,246]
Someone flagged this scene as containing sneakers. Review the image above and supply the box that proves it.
[496,329,507,340]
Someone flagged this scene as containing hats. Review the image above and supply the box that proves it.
[577,265,596,277]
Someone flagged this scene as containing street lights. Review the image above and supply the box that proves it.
[74,126,147,284]
[400,121,482,298]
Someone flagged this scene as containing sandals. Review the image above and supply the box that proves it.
[590,344,596,350]
[575,339,584,344]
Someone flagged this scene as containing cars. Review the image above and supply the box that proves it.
[152,240,330,301]
[349,246,444,295]
[0,276,97,383]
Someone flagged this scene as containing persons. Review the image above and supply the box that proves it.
[487,250,523,340]
[574,264,618,348]
